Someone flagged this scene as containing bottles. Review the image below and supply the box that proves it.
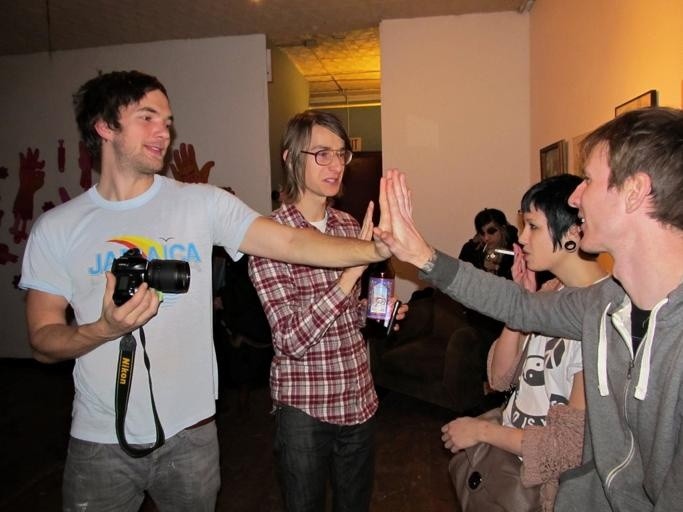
[364,256,397,341]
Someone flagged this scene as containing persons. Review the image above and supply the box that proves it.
[14,70,397,511]
[166,140,217,185]
[442,174,613,510]
[381,108,683,510]
[249,110,409,511]
[458,207,524,395]
[12,147,47,222]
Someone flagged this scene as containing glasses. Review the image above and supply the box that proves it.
[296,147,354,166]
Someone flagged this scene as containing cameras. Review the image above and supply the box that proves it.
[110,247,191,309]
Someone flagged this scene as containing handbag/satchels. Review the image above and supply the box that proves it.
[446,406,542,512]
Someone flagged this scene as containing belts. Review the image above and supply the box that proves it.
[183,414,216,430]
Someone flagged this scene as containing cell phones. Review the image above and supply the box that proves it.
[385,300,401,337]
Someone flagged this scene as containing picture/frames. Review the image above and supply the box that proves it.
[612,88,658,118]
[538,139,570,183]
